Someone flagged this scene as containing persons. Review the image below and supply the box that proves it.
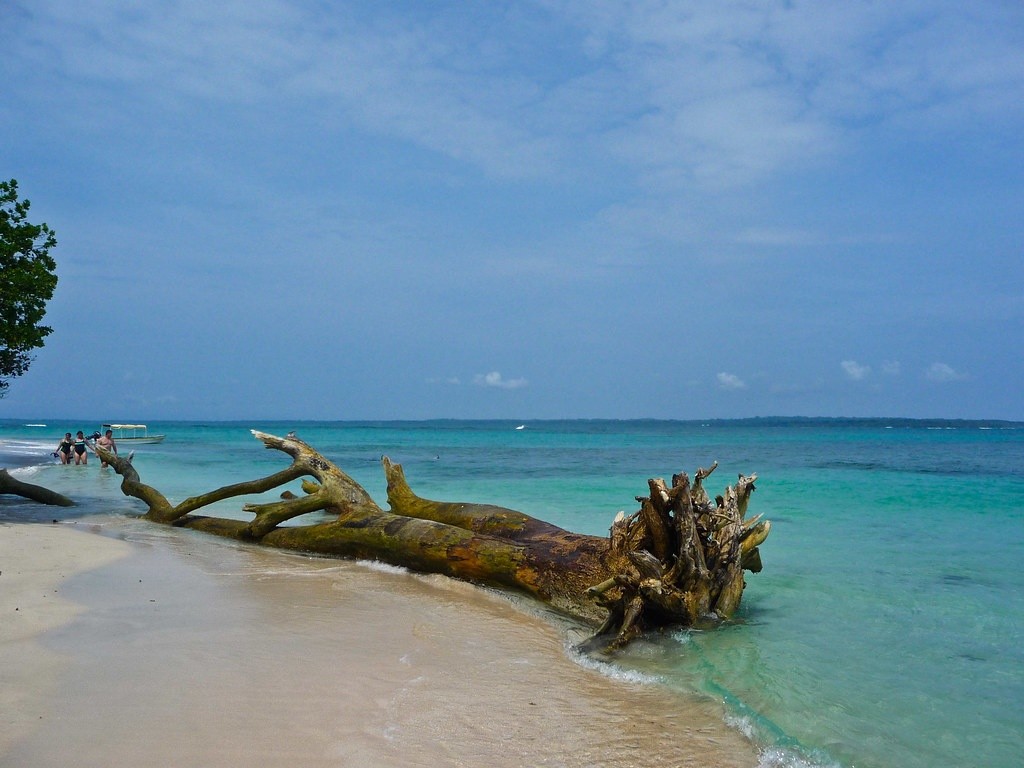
[54,431,76,465]
[71,430,97,465]
[95,429,119,468]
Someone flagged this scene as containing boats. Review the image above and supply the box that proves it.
[88,424,165,447]
